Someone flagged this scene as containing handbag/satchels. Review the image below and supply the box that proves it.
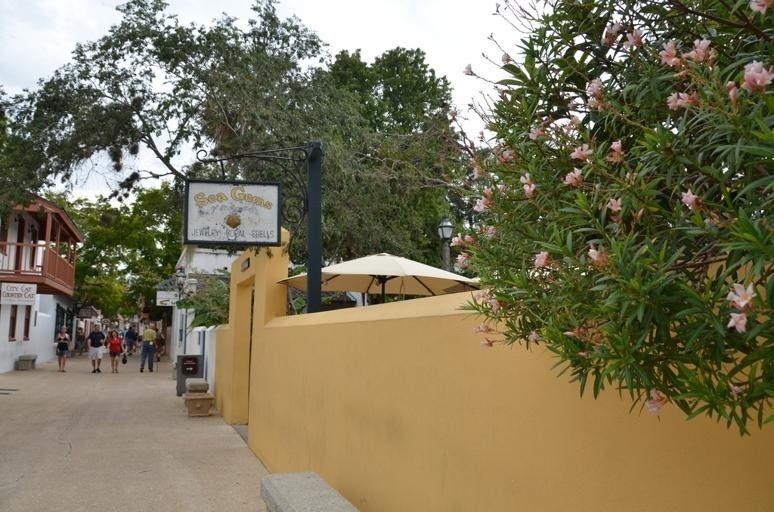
[122,353,128,365]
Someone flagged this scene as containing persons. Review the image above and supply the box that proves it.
[138,321,157,373]
[124,325,135,356]
[54,324,71,373]
[105,330,125,373]
[73,326,85,355]
[83,324,106,373]
[154,325,165,363]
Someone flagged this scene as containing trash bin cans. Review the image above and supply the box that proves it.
[177,355,204,396]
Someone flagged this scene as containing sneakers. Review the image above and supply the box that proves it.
[57,368,67,373]
[140,367,154,373]
[112,368,119,375]
[92,368,102,373]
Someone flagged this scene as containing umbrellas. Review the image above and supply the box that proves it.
[275,252,479,303]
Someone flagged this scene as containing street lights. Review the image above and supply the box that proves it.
[434,213,454,270]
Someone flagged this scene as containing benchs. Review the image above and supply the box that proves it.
[259,469,362,511]
[15,352,37,370]
